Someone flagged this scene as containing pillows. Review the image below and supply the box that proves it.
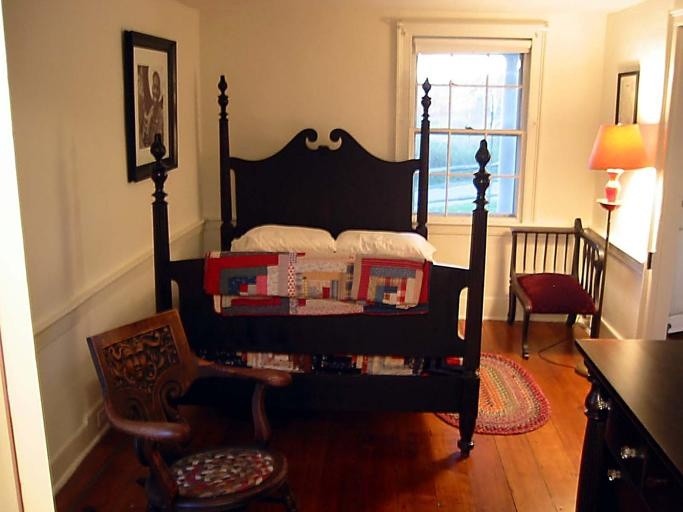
[230,224,336,253]
[338,229,434,262]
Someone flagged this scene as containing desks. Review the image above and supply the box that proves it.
[573,338,683,512]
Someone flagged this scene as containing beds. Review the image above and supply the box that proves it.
[150,75,492,461]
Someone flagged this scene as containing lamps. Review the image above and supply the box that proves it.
[575,123,646,376]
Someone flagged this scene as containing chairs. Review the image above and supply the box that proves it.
[507,218,607,361]
[85,307,292,511]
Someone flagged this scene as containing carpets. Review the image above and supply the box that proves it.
[435,351,551,436]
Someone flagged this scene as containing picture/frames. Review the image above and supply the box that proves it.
[615,70,639,124]
[123,30,178,183]
